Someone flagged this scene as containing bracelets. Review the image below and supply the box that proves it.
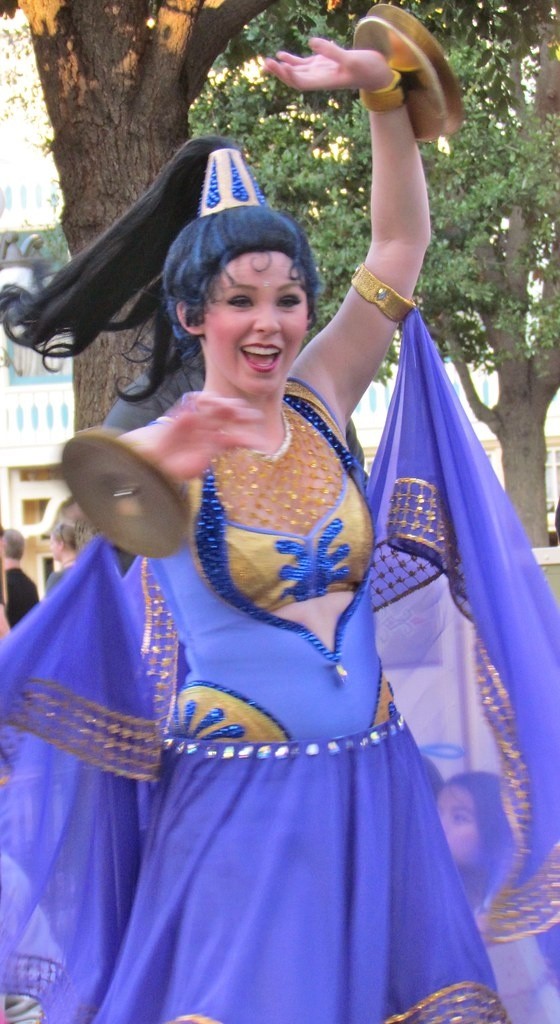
[358,71,408,112]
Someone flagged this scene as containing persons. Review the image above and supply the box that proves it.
[1,528,39,627]
[437,770,560,1024]
[1,38,560,1024]
[45,523,81,597]
[101,331,207,464]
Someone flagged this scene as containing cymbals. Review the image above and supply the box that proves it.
[62,434,192,559]
[350,4,467,142]
